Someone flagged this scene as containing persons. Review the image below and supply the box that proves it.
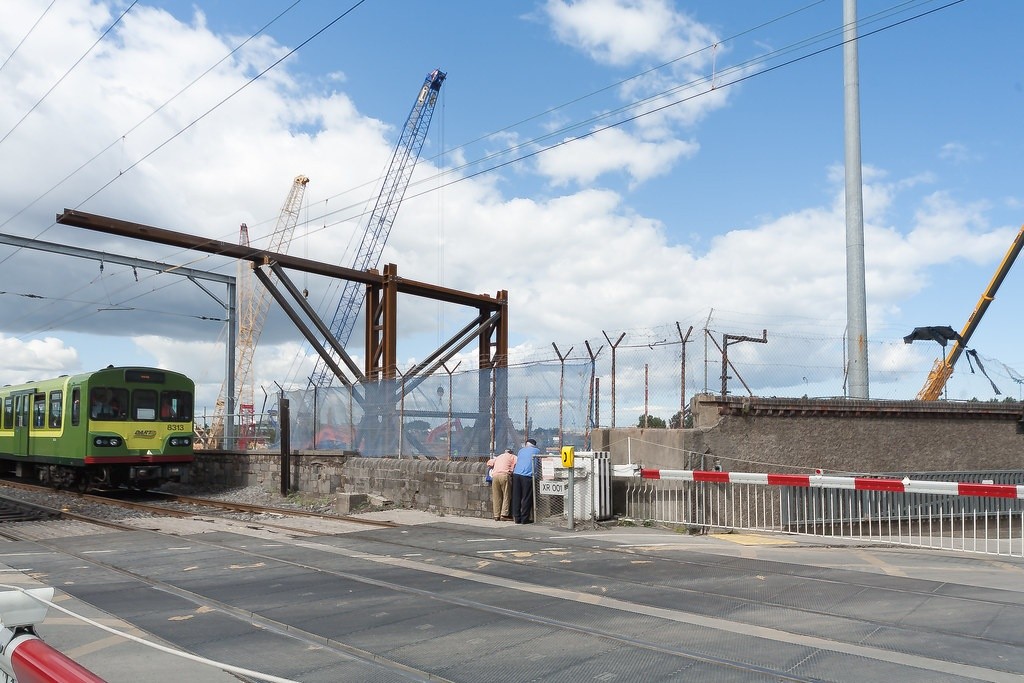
[487,449,518,521]
[90,393,115,417]
[160,396,177,418]
[511,439,542,524]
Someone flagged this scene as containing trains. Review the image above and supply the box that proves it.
[0,364,196,494]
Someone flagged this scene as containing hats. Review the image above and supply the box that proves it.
[526,439,537,445]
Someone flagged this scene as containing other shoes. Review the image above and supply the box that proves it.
[500,515,514,521]
[521,520,534,524]
[515,521,521,524]
[494,517,499,521]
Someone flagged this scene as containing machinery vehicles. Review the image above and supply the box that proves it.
[194,173,309,450]
[285,69,452,453]
[425,418,466,457]
[915,223,1024,399]
